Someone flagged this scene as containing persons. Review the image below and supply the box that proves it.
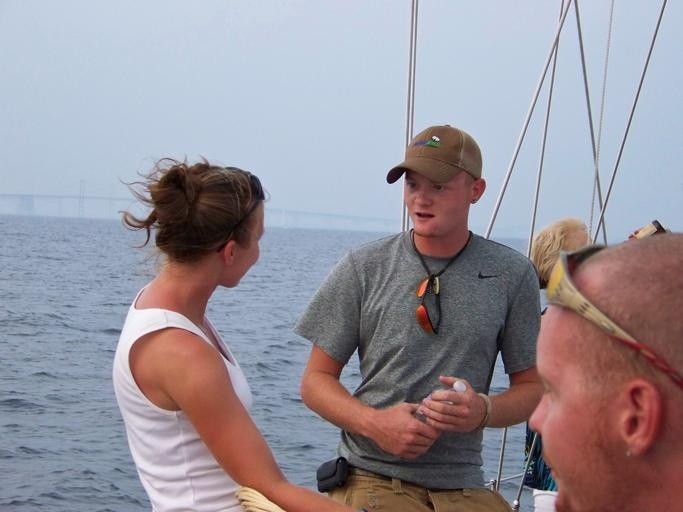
[529,233,683,512]
[518,217,595,512]
[293,123,542,512]
[110,158,352,512]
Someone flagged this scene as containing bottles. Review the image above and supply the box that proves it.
[412,380,467,425]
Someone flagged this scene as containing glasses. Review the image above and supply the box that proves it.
[547,245,681,387]
[416,275,441,335]
[217,166,264,253]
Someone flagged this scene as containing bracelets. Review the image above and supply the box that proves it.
[476,391,492,431]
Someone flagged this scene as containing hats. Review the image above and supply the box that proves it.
[387,124,482,182]
